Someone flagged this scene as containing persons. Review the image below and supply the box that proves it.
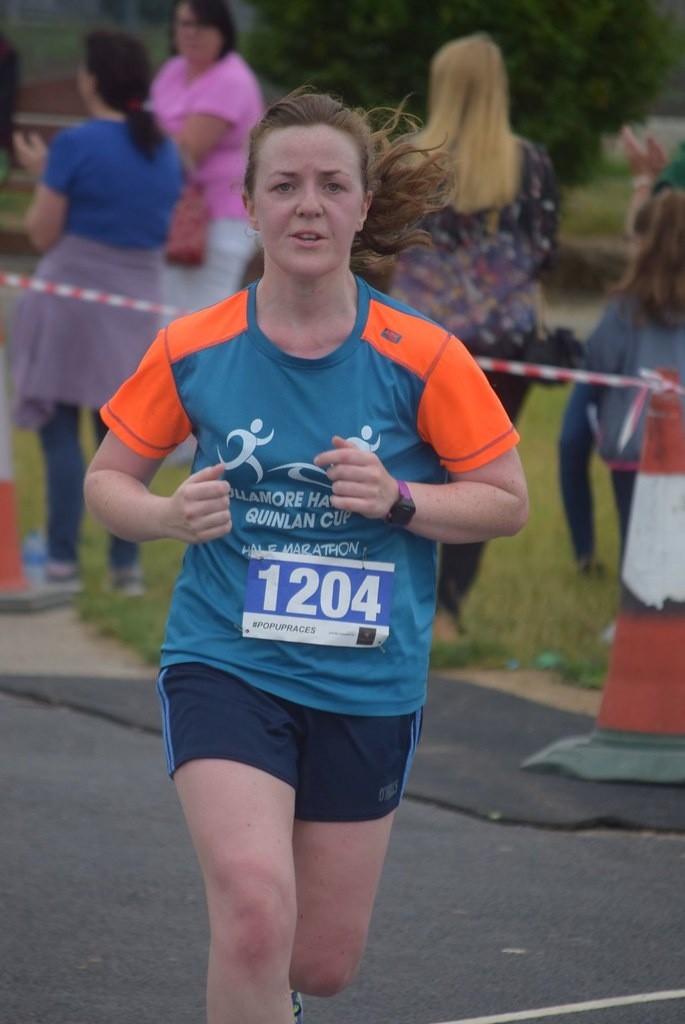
[146,1,267,464]
[620,124,685,237]
[13,31,188,602]
[558,199,685,574]
[379,38,556,642]
[84,95,527,1024]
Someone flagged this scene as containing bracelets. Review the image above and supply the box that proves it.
[633,176,654,188]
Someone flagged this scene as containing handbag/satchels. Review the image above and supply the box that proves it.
[167,183,205,266]
[527,327,584,387]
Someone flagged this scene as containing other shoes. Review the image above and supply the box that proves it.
[46,560,79,593]
[106,567,145,597]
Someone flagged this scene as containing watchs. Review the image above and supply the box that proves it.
[385,482,416,527]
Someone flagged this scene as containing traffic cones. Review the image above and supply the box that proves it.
[520,364,684,791]
[1,321,71,615]
